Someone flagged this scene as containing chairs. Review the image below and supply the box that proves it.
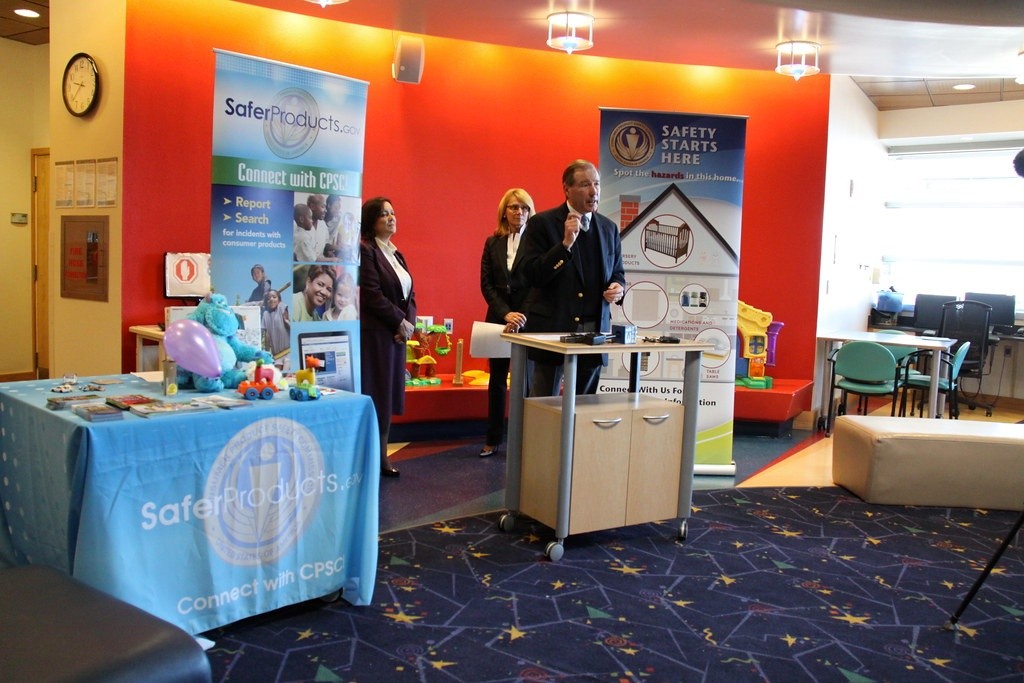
[825,297,1000,431]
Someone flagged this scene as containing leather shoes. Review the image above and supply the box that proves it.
[381,468,400,477]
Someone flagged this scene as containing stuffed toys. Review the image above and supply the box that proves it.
[174,293,274,392]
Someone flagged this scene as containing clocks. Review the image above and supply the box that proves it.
[62,53,100,116]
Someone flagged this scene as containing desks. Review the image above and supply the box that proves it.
[0,371,375,609]
[817,327,958,429]
[128,324,166,371]
[391,372,566,422]
[498,332,719,563]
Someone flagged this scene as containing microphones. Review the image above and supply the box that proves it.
[584,324,638,345]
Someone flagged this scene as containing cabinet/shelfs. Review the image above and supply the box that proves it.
[520,393,686,535]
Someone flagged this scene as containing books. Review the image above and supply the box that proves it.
[47,393,252,422]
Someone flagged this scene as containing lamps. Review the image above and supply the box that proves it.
[776,41,822,81]
[546,12,593,56]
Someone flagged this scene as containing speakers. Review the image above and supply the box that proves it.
[395,36,425,84]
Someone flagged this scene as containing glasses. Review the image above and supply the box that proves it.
[506,204,529,212]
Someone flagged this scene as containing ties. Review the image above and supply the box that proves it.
[581,216,589,231]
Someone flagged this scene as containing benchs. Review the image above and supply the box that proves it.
[832,415,1024,509]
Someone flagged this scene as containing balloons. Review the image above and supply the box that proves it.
[164,317,220,378]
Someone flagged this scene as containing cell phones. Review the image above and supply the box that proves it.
[298,330,355,393]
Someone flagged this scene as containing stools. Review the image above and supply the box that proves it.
[0,566,210,683]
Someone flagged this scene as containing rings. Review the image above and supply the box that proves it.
[510,328,515,332]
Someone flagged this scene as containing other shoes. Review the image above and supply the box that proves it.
[479,445,499,456]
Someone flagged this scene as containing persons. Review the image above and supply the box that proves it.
[359,197,417,475]
[479,188,537,456]
[520,160,626,398]
[247,194,361,366]
